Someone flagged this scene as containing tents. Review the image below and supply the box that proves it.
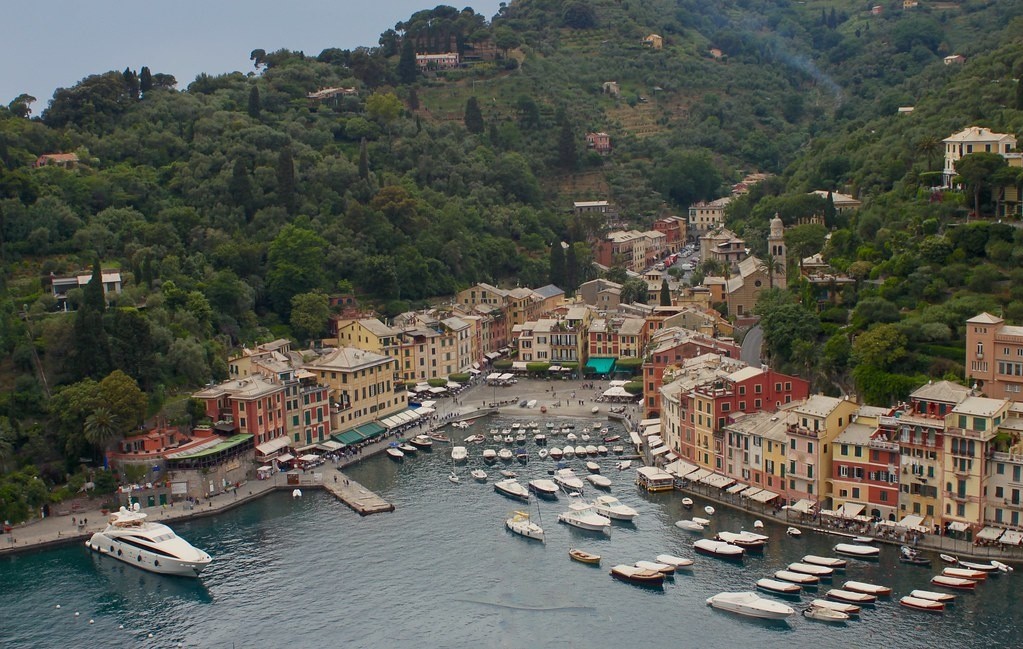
[487,372,502,386]
[602,386,634,409]
[498,373,514,387]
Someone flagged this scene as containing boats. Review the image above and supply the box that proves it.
[899,595,946,611]
[989,560,1013,573]
[489,405,958,622]
[426,430,450,442]
[474,434,485,445]
[397,438,418,453]
[609,564,666,586]
[451,446,468,463]
[636,560,676,579]
[482,447,496,462]
[448,473,461,484]
[464,434,475,443]
[569,547,602,565]
[654,554,695,572]
[292,488,302,499]
[410,435,434,447]
[84,489,213,578]
[958,561,996,571]
[469,420,475,425]
[470,468,488,481]
[386,442,404,458]
[458,421,470,429]
[930,575,978,590]
[942,566,988,582]
[452,421,458,429]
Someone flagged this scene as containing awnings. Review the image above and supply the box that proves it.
[406,347,510,397]
[640,418,780,514]
[255,435,291,455]
[782,497,1023,545]
[277,452,294,462]
[316,399,437,453]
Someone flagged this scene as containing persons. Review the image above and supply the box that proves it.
[770,499,926,543]
[302,410,461,471]
[71,516,75,523]
[80,517,88,525]
[169,488,238,509]
[571,380,597,405]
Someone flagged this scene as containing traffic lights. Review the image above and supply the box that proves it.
[909,589,956,602]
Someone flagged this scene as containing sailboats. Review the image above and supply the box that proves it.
[505,441,547,543]
